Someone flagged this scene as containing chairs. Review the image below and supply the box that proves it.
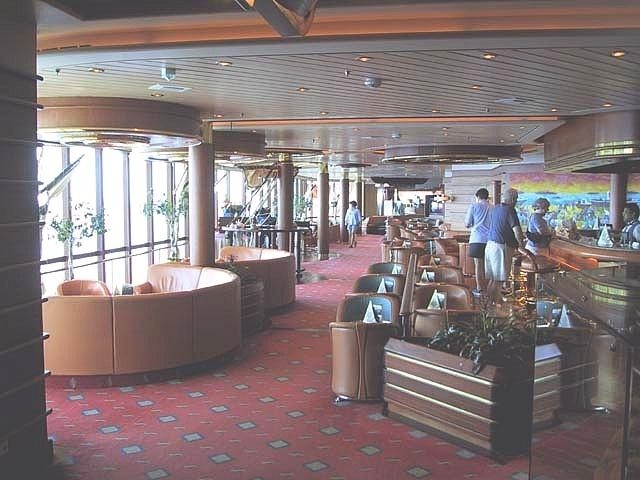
[58,280,110,298]
[329,213,560,407]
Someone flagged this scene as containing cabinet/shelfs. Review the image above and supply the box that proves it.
[528,270,640,480]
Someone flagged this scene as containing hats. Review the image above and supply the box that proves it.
[349,200,358,206]
[531,198,550,207]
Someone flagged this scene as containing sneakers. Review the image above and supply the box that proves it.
[471,286,484,294]
[347,241,359,249]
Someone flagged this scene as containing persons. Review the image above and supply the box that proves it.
[221,203,272,248]
[525,197,555,257]
[617,202,640,251]
[465,187,495,296]
[547,211,557,229]
[483,188,524,308]
[344,201,363,248]
[516,206,528,226]
[583,208,597,229]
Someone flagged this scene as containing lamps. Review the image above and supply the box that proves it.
[159,67,176,82]
[364,76,381,90]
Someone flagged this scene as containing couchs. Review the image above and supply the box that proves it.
[41,263,241,389]
[213,244,297,309]
